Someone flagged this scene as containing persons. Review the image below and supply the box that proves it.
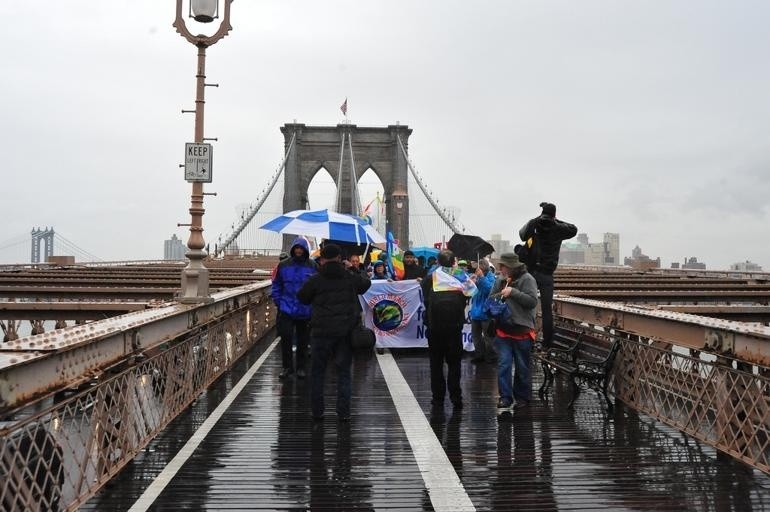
[519,202,577,343]
[297,244,371,420]
[420,251,464,409]
[487,251,539,410]
[271,250,501,378]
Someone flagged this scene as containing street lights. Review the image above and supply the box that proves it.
[170,0,239,310]
[391,183,408,248]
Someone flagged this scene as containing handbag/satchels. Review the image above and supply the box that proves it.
[352,326,377,363]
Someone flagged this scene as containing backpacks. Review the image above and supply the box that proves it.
[481,296,515,341]
[519,220,545,267]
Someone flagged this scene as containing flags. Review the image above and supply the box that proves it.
[340,98,347,116]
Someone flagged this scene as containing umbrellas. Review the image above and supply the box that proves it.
[447,234,495,268]
[409,244,440,258]
[257,210,387,246]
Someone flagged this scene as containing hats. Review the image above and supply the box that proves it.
[319,244,343,260]
[495,251,526,269]
[457,259,468,265]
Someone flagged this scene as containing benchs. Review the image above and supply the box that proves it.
[538,332,621,412]
[535,312,594,399]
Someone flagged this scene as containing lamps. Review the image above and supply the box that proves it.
[392,183,408,209]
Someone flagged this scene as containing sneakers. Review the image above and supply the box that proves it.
[496,395,530,412]
[336,412,351,421]
[278,367,311,382]
[470,356,486,364]
[309,407,324,420]
[428,396,465,409]
